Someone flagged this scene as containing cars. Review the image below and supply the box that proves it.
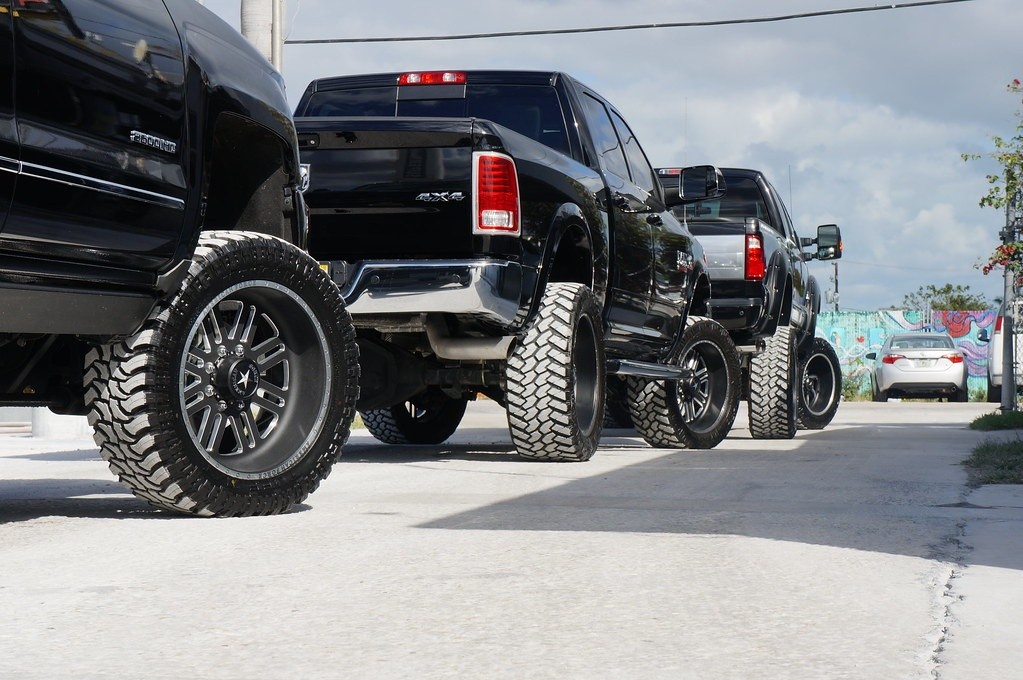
[866,331,969,403]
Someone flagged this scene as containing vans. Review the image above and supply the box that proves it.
[976,297,1022,403]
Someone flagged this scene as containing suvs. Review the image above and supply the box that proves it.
[289,67,742,461]
[1,1,362,520]
[650,165,844,442]
